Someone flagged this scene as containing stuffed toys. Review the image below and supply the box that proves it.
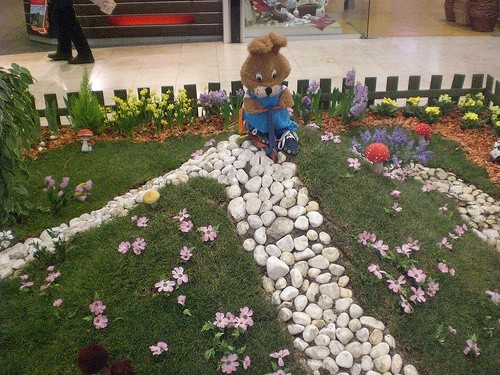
[239,32,303,156]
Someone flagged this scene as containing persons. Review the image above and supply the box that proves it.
[46,0,95,65]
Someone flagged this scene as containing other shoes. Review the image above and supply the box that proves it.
[47,51,72,61]
[68,54,94,64]
